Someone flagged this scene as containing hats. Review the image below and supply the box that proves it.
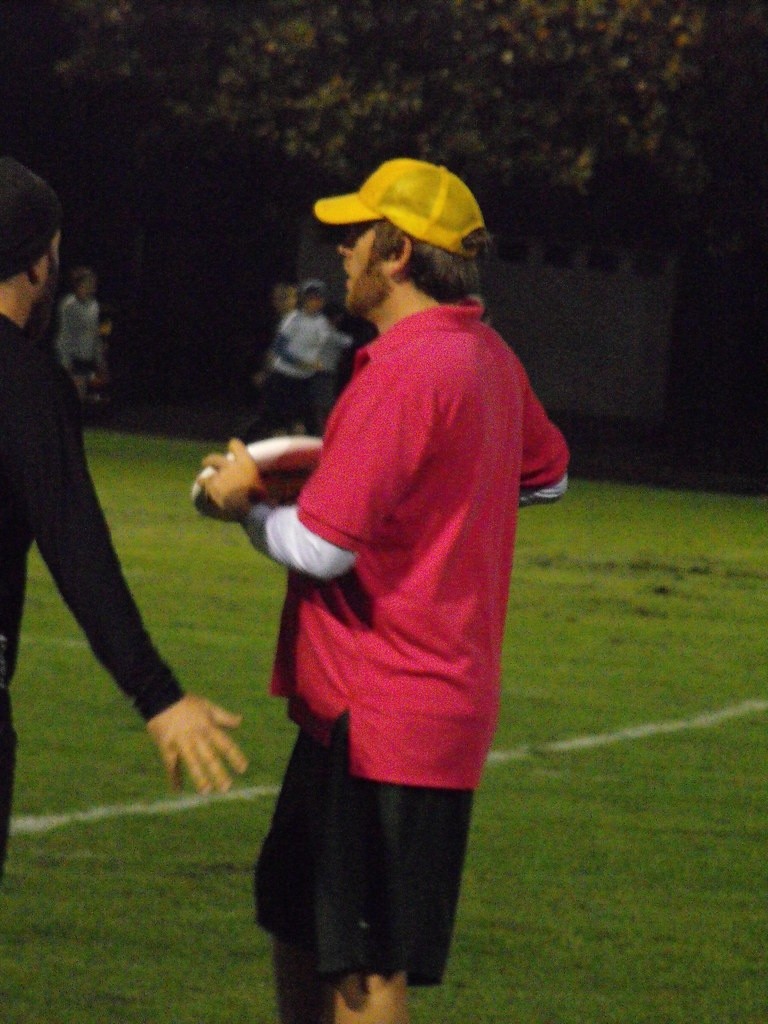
[312,157,486,258]
[0,155,63,280]
[301,276,325,293]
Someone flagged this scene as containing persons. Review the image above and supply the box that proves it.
[238,279,353,444]
[0,148,248,882]
[195,158,567,1024]
[55,0,709,266]
[57,262,111,405]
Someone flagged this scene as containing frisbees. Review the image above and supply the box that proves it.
[189,433,322,517]
